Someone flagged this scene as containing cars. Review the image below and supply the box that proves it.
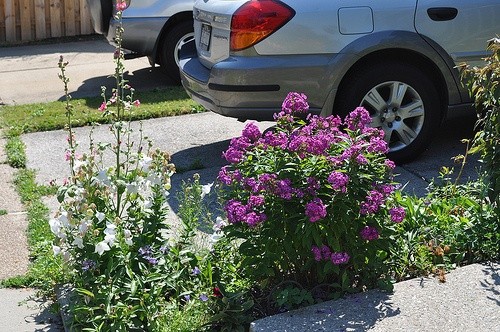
[85,0,197,83]
[175,0,500,166]
